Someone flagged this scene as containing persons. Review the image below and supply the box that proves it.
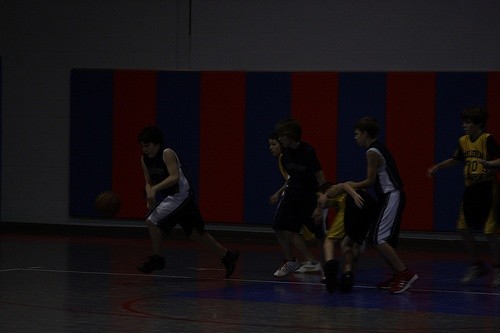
[267,115,421,299]
[427,105,500,289]
[136,128,242,279]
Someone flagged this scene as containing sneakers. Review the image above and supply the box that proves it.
[460,264,486,284]
[296,260,321,272]
[492,272,500,286]
[138,255,166,275]
[379,276,398,290]
[222,248,240,278]
[325,259,338,292]
[343,272,353,286]
[274,258,300,277]
[390,270,419,294]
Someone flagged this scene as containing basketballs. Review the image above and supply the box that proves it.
[96,190,120,217]
